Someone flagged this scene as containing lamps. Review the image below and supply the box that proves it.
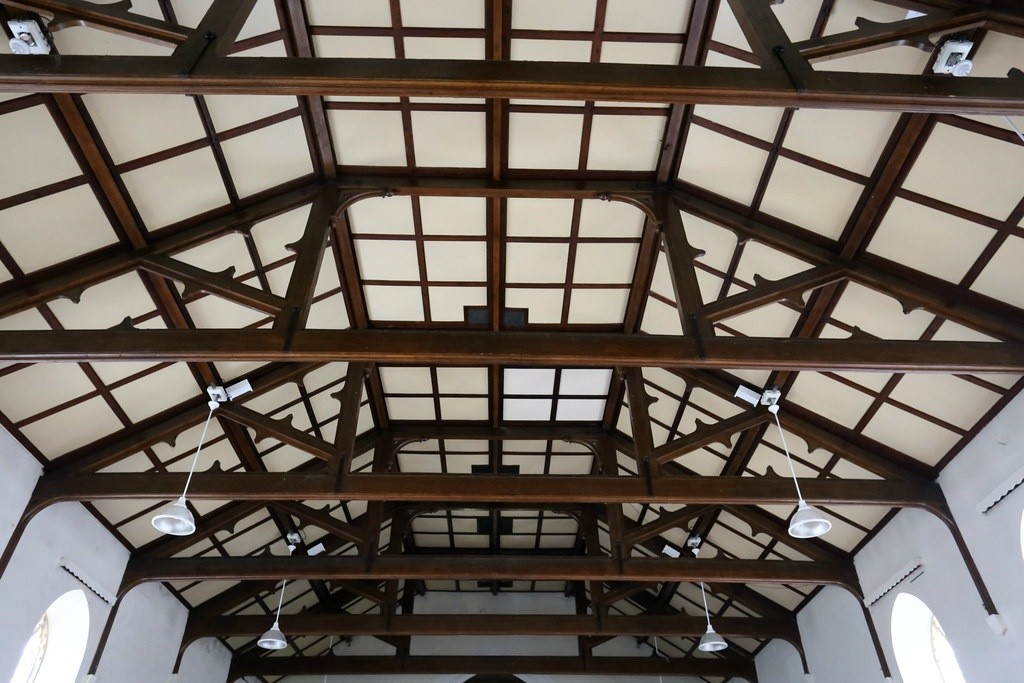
[688,536,728,652]
[151,386,226,537]
[761,389,832,539]
[257,532,302,650]
